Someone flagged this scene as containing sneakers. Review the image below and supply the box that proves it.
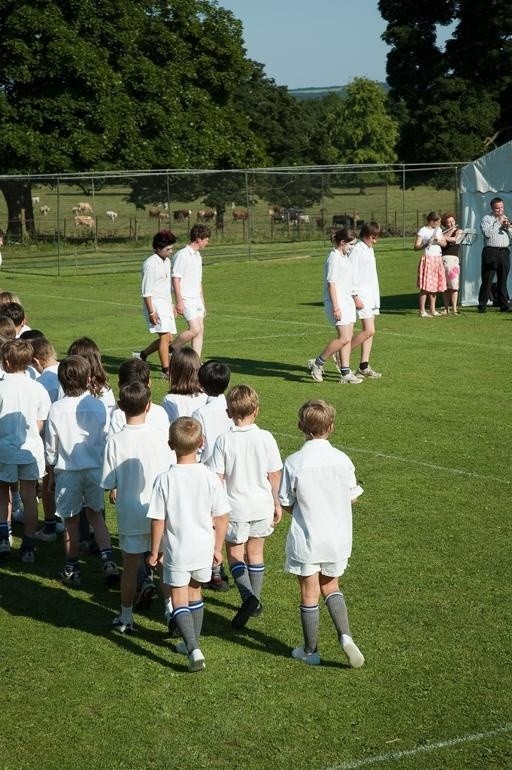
[340,632,365,669]
[292,647,320,666]
[160,369,169,378]
[0,516,120,586]
[231,596,259,630]
[133,577,156,612]
[308,352,382,384]
[479,308,486,313]
[418,311,460,318]
[132,351,145,361]
[113,616,133,633]
[500,307,512,313]
[188,649,205,671]
[166,612,182,638]
[249,604,262,616]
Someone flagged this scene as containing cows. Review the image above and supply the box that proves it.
[106,211,118,221]
[32,196,39,204]
[231,211,248,224]
[74,216,95,228]
[318,218,326,228]
[39,205,50,216]
[333,215,356,228]
[148,209,191,223]
[268,203,310,226]
[196,211,217,223]
[77,203,93,214]
[72,207,81,215]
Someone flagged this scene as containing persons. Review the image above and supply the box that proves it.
[413,211,447,317]
[277,398,367,671]
[98,379,185,638]
[166,224,212,361]
[129,229,178,380]
[189,358,234,591]
[209,382,284,630]
[305,226,363,384]
[438,212,465,316]
[477,197,512,314]
[330,221,384,380]
[145,413,232,673]
[0,291,210,594]
